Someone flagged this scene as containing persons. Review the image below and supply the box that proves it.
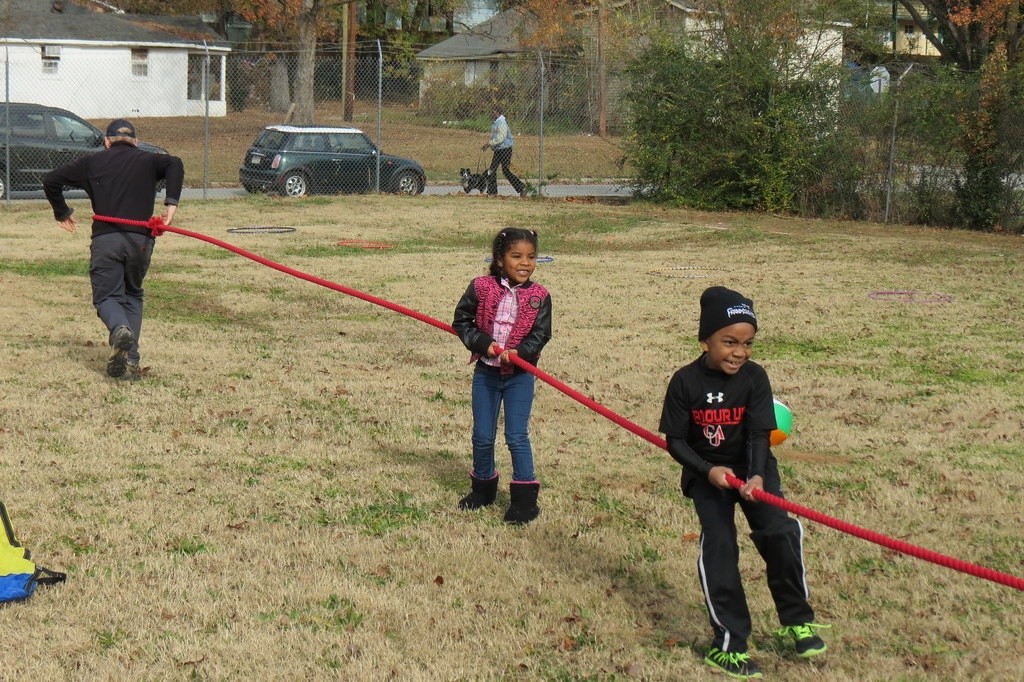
[452,228,552,526]
[45,119,185,379]
[482,107,529,196]
[659,286,826,677]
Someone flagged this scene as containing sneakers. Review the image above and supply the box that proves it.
[704,642,764,680]
[778,622,832,659]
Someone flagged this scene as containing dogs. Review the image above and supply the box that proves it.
[459,167,496,194]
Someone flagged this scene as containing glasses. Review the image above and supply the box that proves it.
[491,111,494,114]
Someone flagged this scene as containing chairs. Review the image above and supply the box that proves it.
[314,137,323,148]
[302,135,312,147]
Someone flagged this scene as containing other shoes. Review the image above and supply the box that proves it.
[105,325,136,378]
[121,354,141,382]
[489,191,498,197]
[519,186,529,198]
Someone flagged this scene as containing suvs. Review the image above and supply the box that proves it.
[238,123,427,197]
[0,101,171,201]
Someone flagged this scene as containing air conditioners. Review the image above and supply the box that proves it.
[41,46,61,58]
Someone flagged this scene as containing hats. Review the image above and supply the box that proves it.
[698,285,758,342]
[493,106,503,114]
[105,119,136,139]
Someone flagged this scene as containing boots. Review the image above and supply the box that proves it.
[502,480,541,527]
[457,467,499,511]
